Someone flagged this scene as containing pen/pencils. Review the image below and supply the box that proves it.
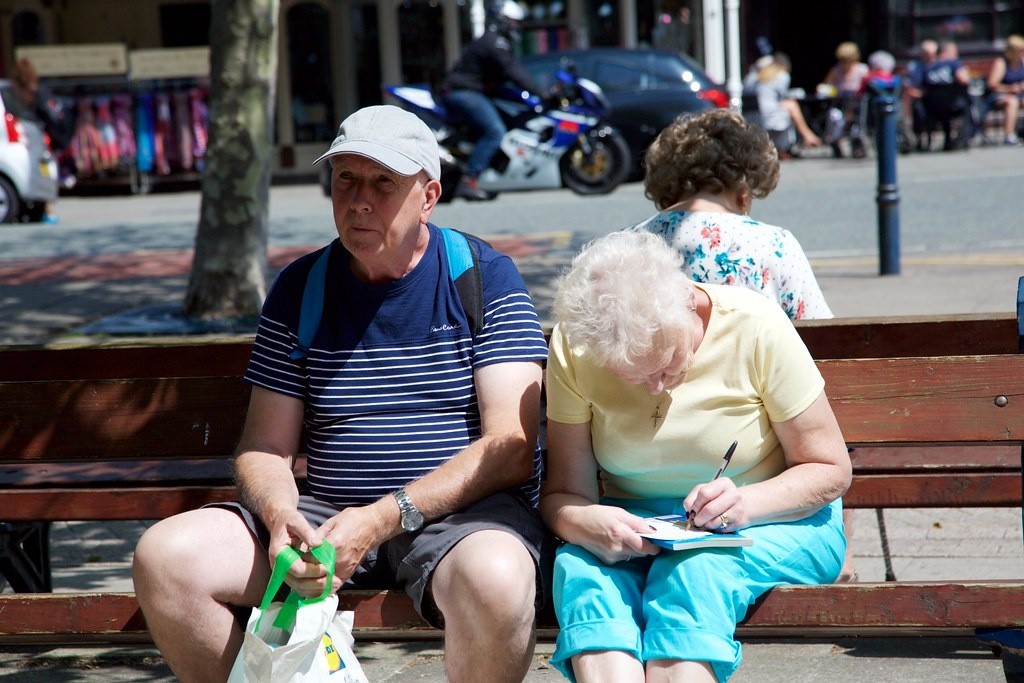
[685,441,739,531]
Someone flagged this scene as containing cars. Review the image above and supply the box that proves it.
[505,46,734,181]
[0,80,60,221]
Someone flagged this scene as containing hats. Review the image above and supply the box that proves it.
[312,104,441,182]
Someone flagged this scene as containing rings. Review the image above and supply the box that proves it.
[626,555,631,561]
[721,514,728,530]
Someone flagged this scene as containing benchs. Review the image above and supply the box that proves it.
[0,313,1024,683]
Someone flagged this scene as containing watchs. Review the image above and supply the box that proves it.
[393,489,423,532]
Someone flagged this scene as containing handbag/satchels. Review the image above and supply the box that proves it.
[227,539,369,683]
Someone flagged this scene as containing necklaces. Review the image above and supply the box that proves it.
[644,385,672,428]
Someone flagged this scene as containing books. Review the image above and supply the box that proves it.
[642,514,752,551]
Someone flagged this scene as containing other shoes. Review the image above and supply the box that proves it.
[777,152,800,160]
[831,149,841,158]
[804,135,822,148]
[1006,132,1020,144]
[853,145,865,158]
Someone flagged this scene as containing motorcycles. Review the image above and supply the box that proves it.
[382,58,634,203]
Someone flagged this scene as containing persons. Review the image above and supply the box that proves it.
[133,104,550,683]
[442,0,550,197]
[744,53,822,159]
[822,39,971,159]
[983,34,1024,144]
[617,109,856,584]
[14,59,38,93]
[538,231,853,683]
